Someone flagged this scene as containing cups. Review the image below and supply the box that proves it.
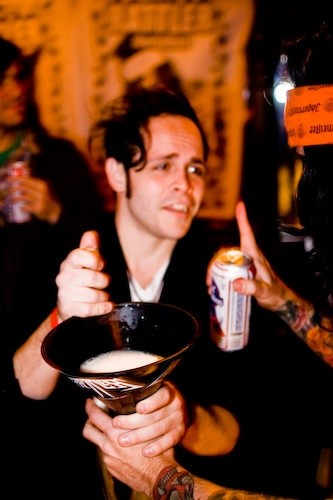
[41,302,199,416]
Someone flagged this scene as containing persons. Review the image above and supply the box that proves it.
[0,36,104,316]
[0,87,285,500]
[80,201,333,500]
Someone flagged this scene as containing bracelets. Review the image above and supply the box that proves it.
[48,308,58,329]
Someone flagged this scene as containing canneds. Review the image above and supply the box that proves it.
[3,161,31,224]
[206,249,253,352]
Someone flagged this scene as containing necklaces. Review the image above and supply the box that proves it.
[127,269,163,303]
